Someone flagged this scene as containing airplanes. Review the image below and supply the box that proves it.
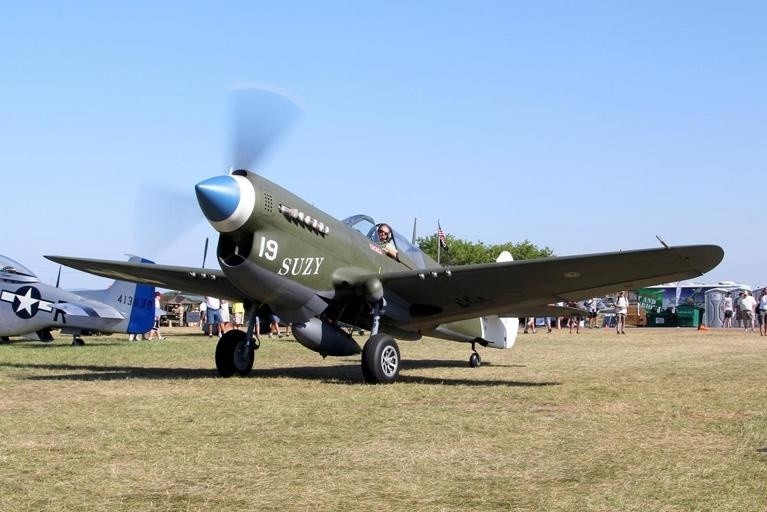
[42,87,725,379]
[0,244,161,350]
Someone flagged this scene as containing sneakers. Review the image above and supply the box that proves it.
[133,336,167,341]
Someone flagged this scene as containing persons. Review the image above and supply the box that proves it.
[612,291,629,333]
[368,224,397,259]
[524,298,599,333]
[128,292,293,341]
[721,287,767,336]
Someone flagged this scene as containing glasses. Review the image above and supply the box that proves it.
[379,228,388,235]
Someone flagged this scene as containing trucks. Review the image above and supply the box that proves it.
[643,279,750,317]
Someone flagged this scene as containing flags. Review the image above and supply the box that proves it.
[438,224,449,251]
